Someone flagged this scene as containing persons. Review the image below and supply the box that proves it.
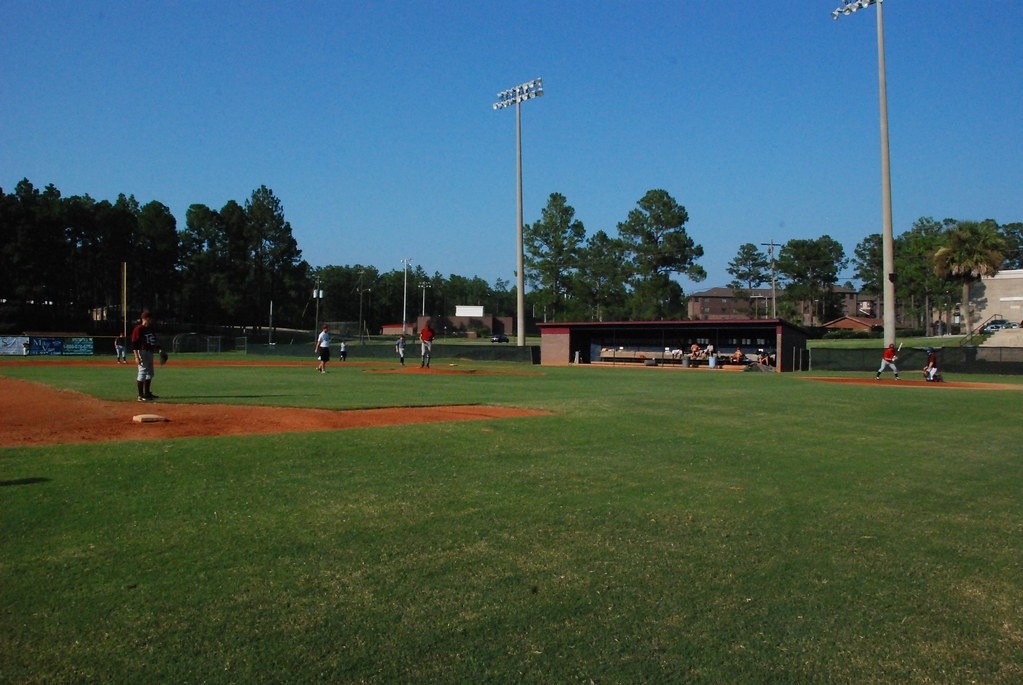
[396,335,406,366]
[923,347,943,382]
[132,312,168,400]
[674,341,770,366]
[315,325,331,373]
[876,344,901,380]
[22,341,30,356]
[339,340,347,361]
[115,333,127,364]
[420,322,435,368]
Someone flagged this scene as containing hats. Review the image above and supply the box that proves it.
[322,325,328,329]
[142,311,154,320]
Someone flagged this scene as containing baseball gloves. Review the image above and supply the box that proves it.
[924,371,929,378]
[159,350,168,365]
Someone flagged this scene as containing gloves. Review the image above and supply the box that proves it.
[891,358,895,362]
[894,356,898,360]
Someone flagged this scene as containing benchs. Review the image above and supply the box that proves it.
[595,346,775,367]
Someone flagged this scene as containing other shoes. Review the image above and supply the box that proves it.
[321,370,326,373]
[117,361,121,364]
[138,396,151,402]
[145,393,158,399]
[895,377,901,380]
[875,377,879,380]
[426,366,429,369]
[318,366,322,372]
[123,360,127,363]
[420,364,424,367]
[400,363,405,366]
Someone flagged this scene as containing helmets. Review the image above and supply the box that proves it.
[927,347,935,353]
[889,344,894,349]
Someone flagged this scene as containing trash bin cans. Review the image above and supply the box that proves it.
[935,321,945,335]
[682,356,690,368]
[709,356,717,368]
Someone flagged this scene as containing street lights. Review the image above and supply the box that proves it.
[359,288,372,344]
[401,258,414,335]
[493,79,543,344]
[831,0,895,350]
[417,281,432,316]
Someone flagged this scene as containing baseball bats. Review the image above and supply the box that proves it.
[892,342,903,362]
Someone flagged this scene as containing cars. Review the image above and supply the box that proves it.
[982,319,1021,333]
[490,335,509,344]
